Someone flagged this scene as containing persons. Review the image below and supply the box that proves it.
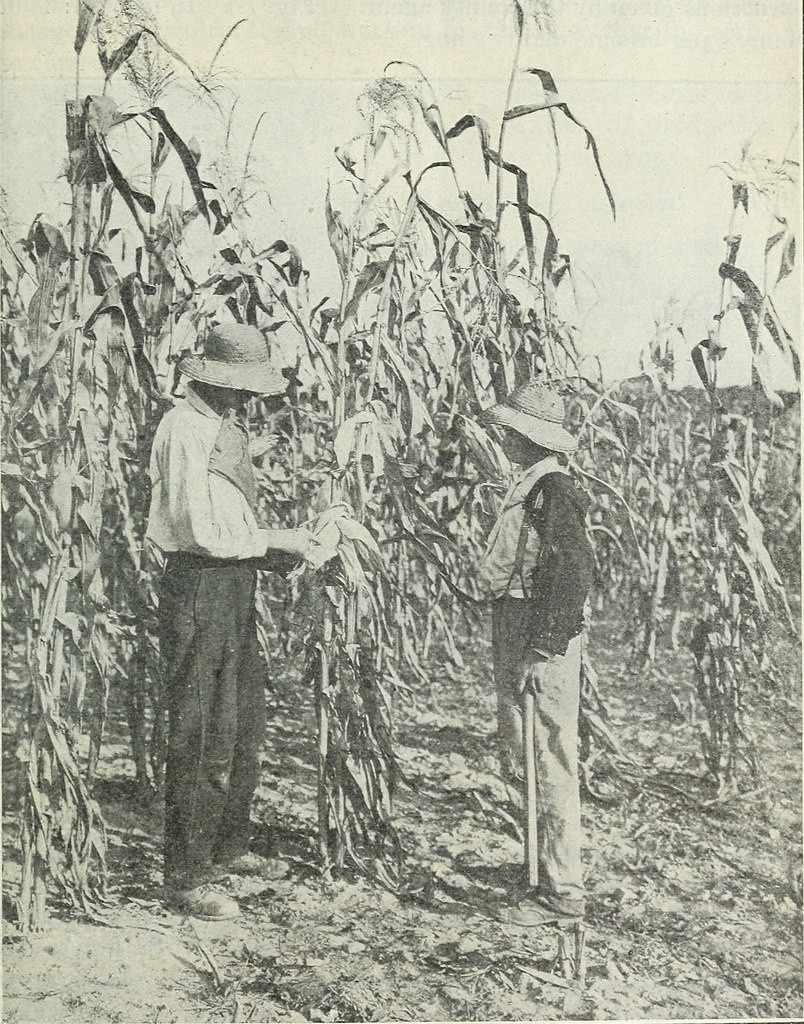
[477,385,595,927]
[146,323,321,922]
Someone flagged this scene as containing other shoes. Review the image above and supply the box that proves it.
[167,882,240,921]
[213,848,291,880]
[498,859,547,885]
[495,896,583,927]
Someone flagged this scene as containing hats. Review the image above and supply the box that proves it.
[179,322,290,394]
[480,383,578,452]
